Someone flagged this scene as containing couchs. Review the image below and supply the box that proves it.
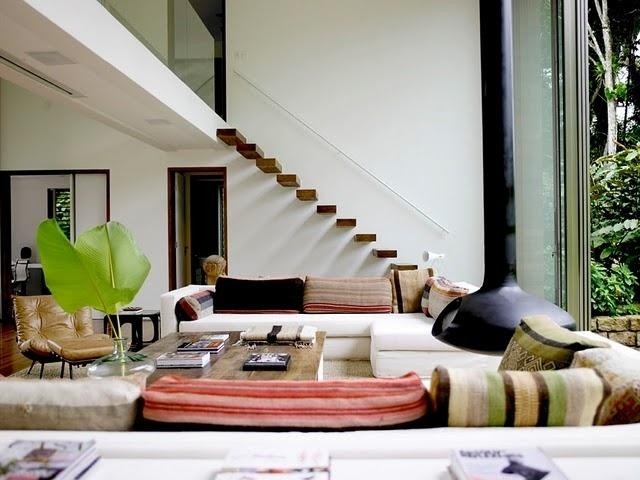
[0,330,640,458]
[161,285,505,378]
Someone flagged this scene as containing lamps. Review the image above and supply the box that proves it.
[423,251,446,272]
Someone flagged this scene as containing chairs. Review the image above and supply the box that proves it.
[10,259,33,296]
[10,295,116,379]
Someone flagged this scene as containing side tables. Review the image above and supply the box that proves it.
[104,310,160,351]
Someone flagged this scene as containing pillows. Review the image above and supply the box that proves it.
[0,366,143,431]
[429,315,640,428]
[142,375,431,430]
[177,269,469,321]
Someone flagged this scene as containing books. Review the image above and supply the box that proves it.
[155,333,229,370]
[437,444,568,480]
[242,352,292,372]
[211,445,333,480]
[0,438,103,480]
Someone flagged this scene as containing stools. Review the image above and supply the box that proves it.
[48,334,114,379]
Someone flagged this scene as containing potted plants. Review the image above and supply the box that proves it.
[35,217,157,380]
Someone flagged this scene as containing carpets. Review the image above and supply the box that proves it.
[7,339,374,383]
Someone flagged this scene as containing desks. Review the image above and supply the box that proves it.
[131,331,327,381]
